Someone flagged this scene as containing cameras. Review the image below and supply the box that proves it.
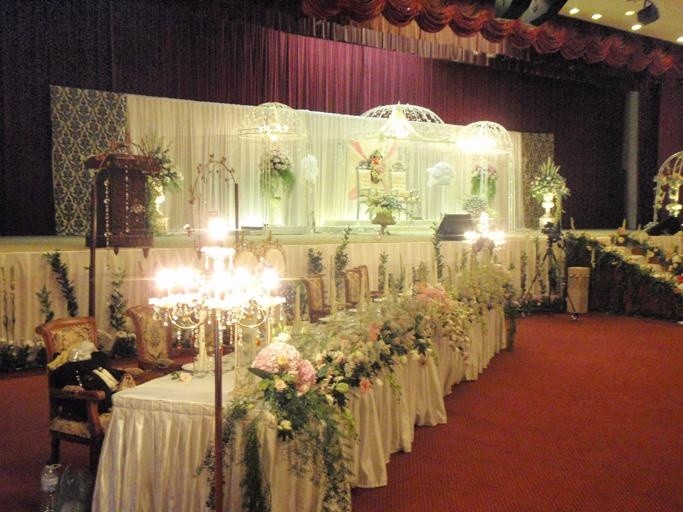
[542,221,560,239]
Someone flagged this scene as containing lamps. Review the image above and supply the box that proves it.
[636,0,660,24]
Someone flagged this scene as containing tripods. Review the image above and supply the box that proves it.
[520,239,580,320]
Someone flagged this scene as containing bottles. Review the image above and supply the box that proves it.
[38,465,59,512]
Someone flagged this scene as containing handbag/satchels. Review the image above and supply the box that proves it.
[56,351,126,419]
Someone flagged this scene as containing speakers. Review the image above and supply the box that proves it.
[437,214,473,241]
